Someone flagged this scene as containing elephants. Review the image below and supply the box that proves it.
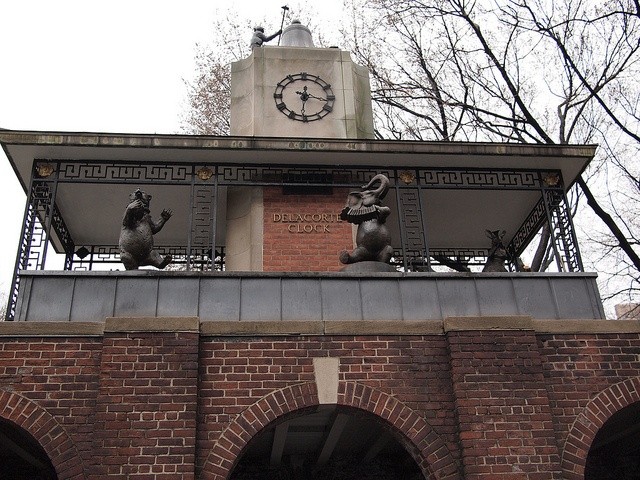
[338,173,395,265]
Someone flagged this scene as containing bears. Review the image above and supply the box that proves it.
[118,186,173,271]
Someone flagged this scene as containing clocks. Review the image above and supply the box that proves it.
[274,71,334,122]
